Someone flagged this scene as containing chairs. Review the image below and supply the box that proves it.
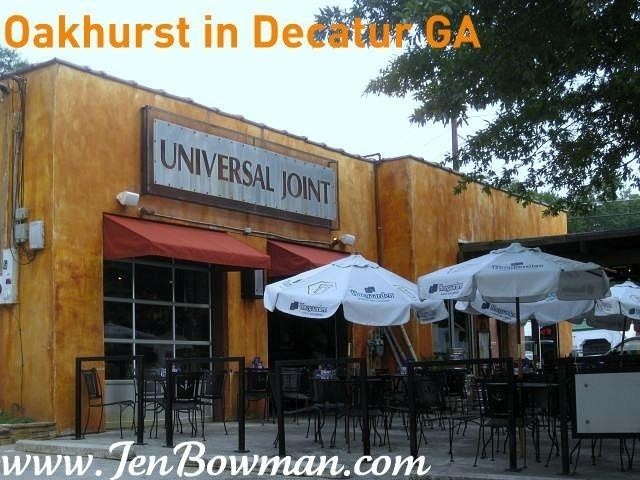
[78,354,640,478]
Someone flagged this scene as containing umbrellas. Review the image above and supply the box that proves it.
[417,242,613,376]
[454,287,595,358]
[564,278,640,355]
[263,249,450,402]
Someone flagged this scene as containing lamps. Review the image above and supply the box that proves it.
[115,190,141,208]
[339,233,357,247]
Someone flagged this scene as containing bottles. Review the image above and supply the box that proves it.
[252,357,263,372]
[400,366,409,376]
[160,362,178,378]
[315,360,337,380]
